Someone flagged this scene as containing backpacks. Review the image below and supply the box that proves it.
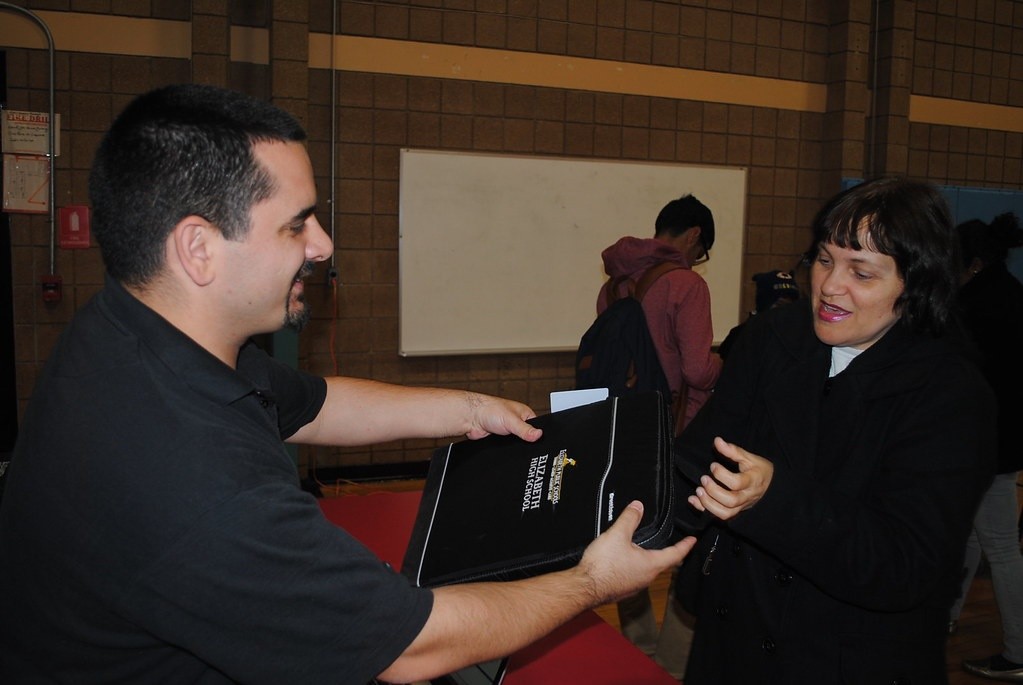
[574,260,688,438]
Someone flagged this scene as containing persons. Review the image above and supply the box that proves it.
[663,173,994,685]
[0,84,697,685]
[596,195,722,680]
[750,269,800,313]
[940,209,1023,684]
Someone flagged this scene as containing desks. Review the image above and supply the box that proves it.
[321,489,680,685]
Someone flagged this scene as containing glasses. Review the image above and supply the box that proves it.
[691,236,709,266]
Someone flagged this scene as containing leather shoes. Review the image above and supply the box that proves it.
[962,655,1023,683]
[948,620,957,635]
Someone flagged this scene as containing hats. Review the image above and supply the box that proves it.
[753,269,800,313]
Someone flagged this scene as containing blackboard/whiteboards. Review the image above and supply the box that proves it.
[398,149,748,357]
